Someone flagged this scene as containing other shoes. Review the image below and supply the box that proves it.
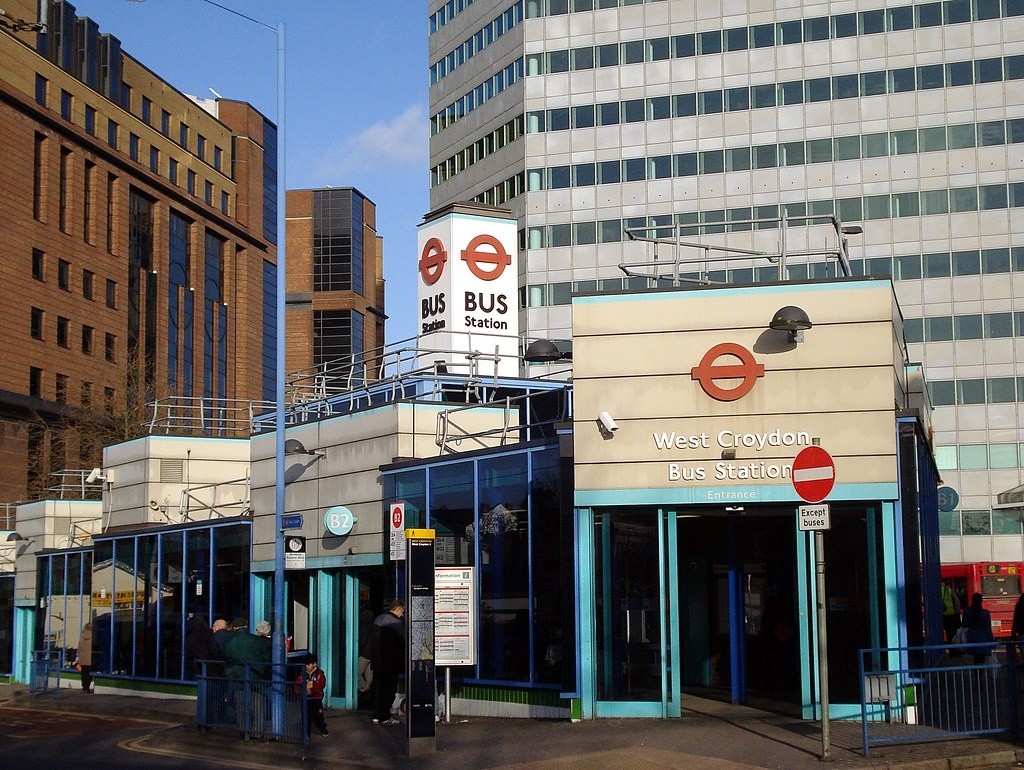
[372,718,381,724]
[322,731,329,737]
[82,690,90,694]
[382,717,401,725]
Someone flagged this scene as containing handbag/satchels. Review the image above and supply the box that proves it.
[983,649,999,679]
[951,627,969,650]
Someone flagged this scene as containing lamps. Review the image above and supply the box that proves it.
[769,306,813,343]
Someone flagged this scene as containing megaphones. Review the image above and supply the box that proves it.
[149,500,159,509]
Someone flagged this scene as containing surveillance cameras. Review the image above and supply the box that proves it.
[599,411,619,432]
[85,468,101,483]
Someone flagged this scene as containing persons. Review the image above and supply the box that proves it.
[292,654,331,737]
[77,622,91,695]
[202,618,273,737]
[369,599,440,725]
[942,579,1024,692]
